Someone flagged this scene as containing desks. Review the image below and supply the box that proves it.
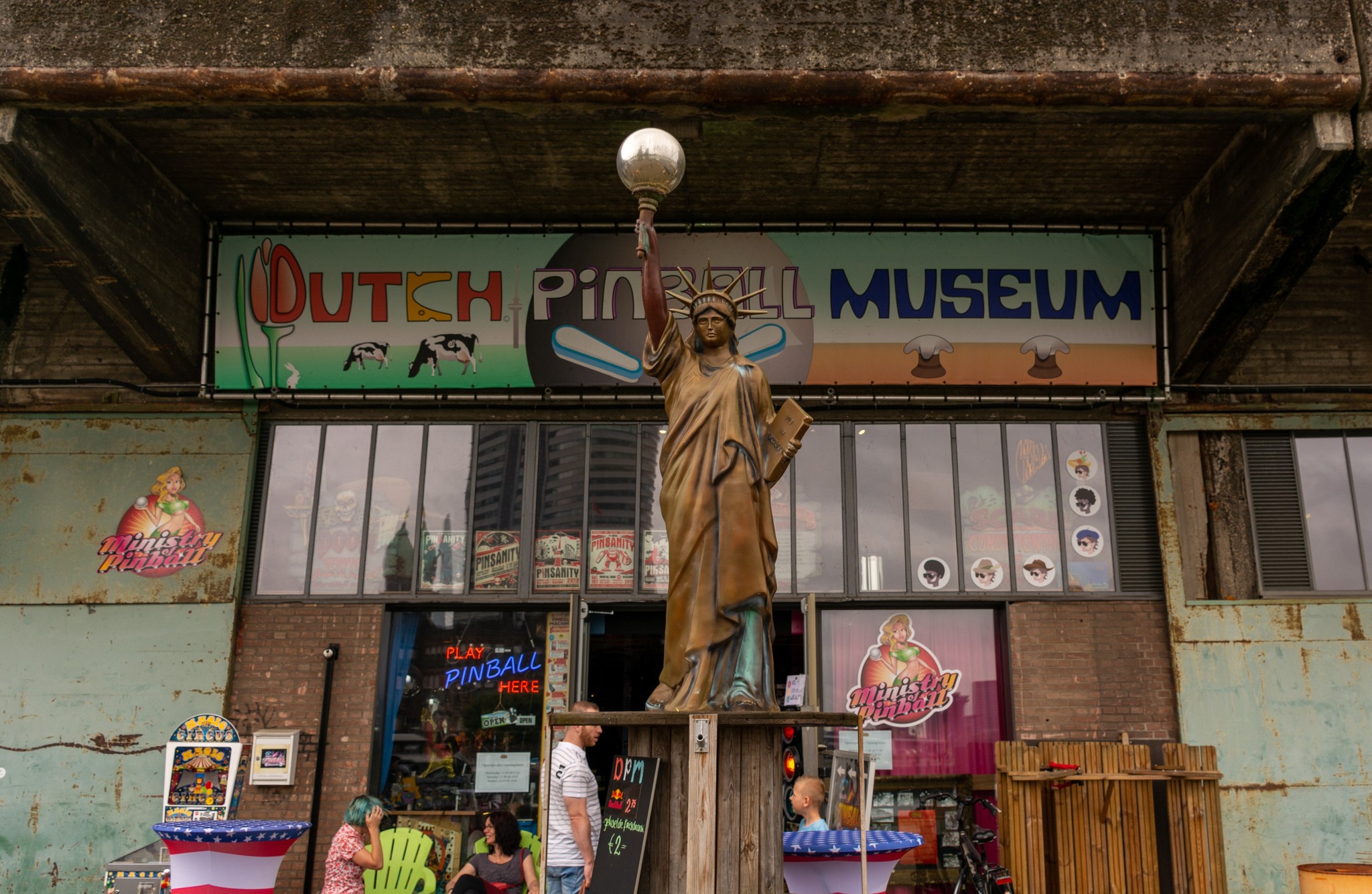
[781,829,922,894]
[154,821,310,893]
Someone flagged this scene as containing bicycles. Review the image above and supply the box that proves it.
[919,793,1016,894]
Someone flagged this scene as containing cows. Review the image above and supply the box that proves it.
[408,333,483,378]
[343,342,393,370]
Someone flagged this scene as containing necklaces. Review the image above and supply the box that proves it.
[496,860,499,864]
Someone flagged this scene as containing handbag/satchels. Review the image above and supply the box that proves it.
[480,876,508,894]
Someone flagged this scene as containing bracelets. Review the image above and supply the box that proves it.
[530,885,539,891]
[447,888,453,894]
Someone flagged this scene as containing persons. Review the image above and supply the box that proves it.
[445,810,540,894]
[320,794,392,894]
[634,218,813,707]
[789,776,830,832]
[427,710,456,761]
[540,701,602,894]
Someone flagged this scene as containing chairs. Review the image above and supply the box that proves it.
[475,831,541,893]
[363,829,439,894]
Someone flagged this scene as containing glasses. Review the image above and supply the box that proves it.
[483,823,495,831]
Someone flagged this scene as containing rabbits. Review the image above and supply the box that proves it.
[284,363,300,388]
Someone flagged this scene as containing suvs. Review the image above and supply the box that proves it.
[391,733,451,779]
